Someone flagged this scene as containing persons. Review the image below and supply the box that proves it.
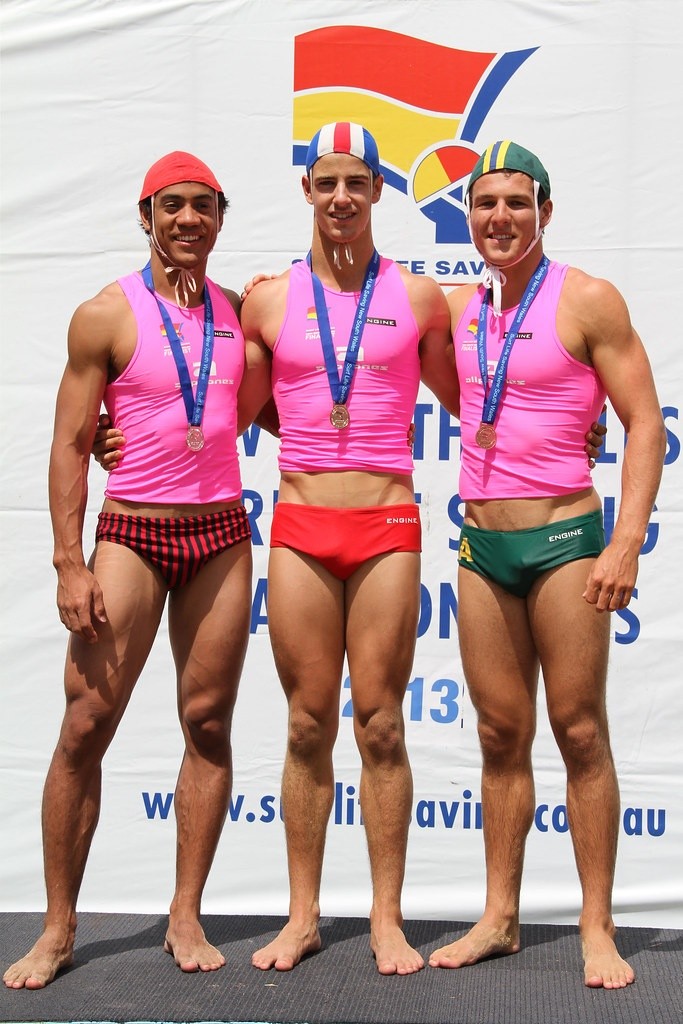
[90,119,607,975]
[238,140,666,988]
[0,146,420,990]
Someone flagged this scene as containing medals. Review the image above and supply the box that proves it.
[185,425,205,452]
[475,421,497,450]
[328,403,350,429]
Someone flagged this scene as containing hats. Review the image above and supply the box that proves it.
[465,139,552,199]
[136,149,224,203]
[305,121,380,177]
